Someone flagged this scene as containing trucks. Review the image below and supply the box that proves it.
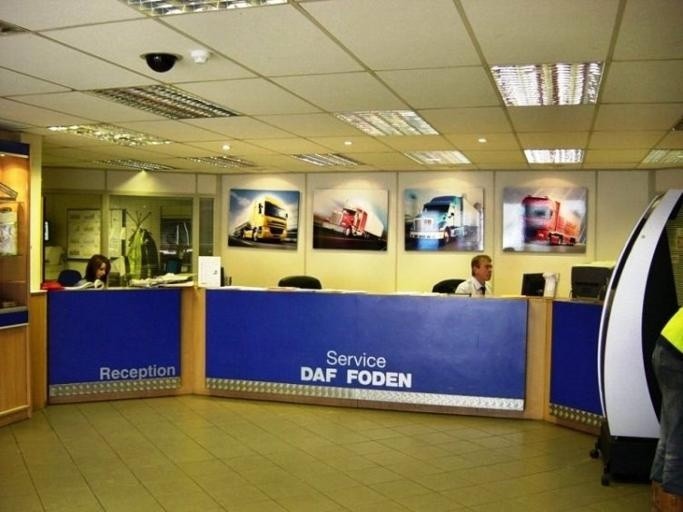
[234,196,288,241]
[522,195,578,245]
[322,208,384,238]
[409,196,480,243]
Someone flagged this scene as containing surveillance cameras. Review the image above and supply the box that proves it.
[146,54,177,73]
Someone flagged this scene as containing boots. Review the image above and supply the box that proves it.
[651,480,683,511]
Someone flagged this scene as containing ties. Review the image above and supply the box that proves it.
[481,287,486,295]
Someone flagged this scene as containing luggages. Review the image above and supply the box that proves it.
[590,418,659,486]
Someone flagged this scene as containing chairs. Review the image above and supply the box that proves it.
[58,269,83,287]
[278,276,321,290]
[432,279,466,294]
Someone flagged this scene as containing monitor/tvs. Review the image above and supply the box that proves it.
[522,272,545,296]
[571,266,613,302]
[166,258,181,274]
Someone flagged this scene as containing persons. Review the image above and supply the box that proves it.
[453,254,497,299]
[73,254,111,290]
[649,307,683,495]
